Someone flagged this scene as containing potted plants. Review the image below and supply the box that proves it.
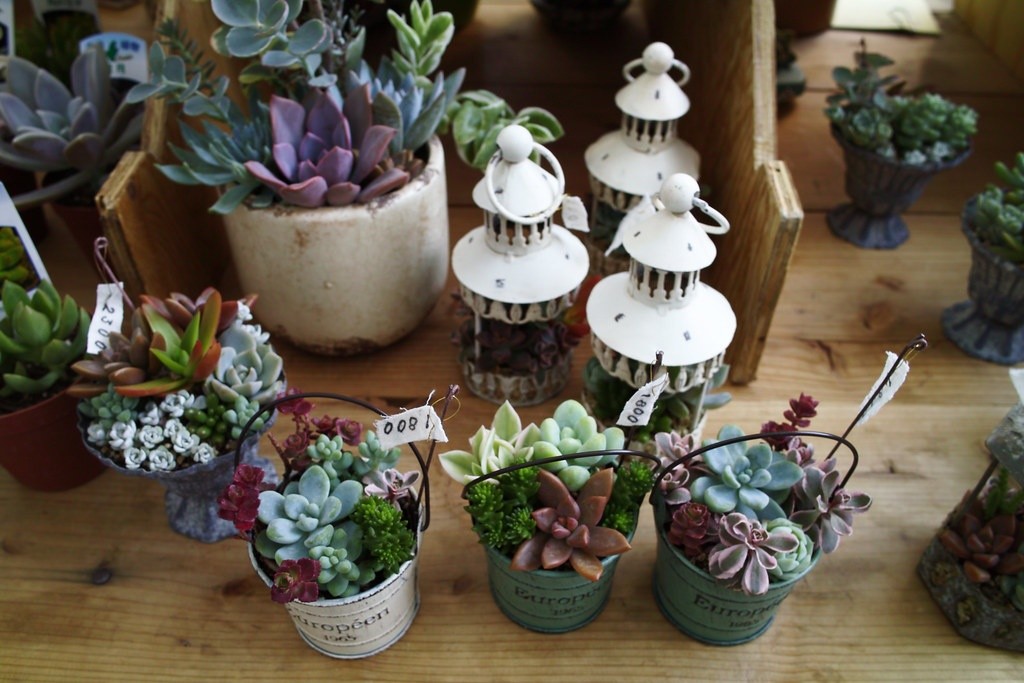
[583,42,701,280]
[916,401,1023,652]
[126,1,465,355]
[452,125,590,402]
[218,384,461,659]
[648,334,926,645]
[824,39,978,249]
[438,350,669,634]
[0,42,143,278]
[66,237,286,542]
[585,173,737,458]
[941,152,1024,363]
[0,278,110,492]
